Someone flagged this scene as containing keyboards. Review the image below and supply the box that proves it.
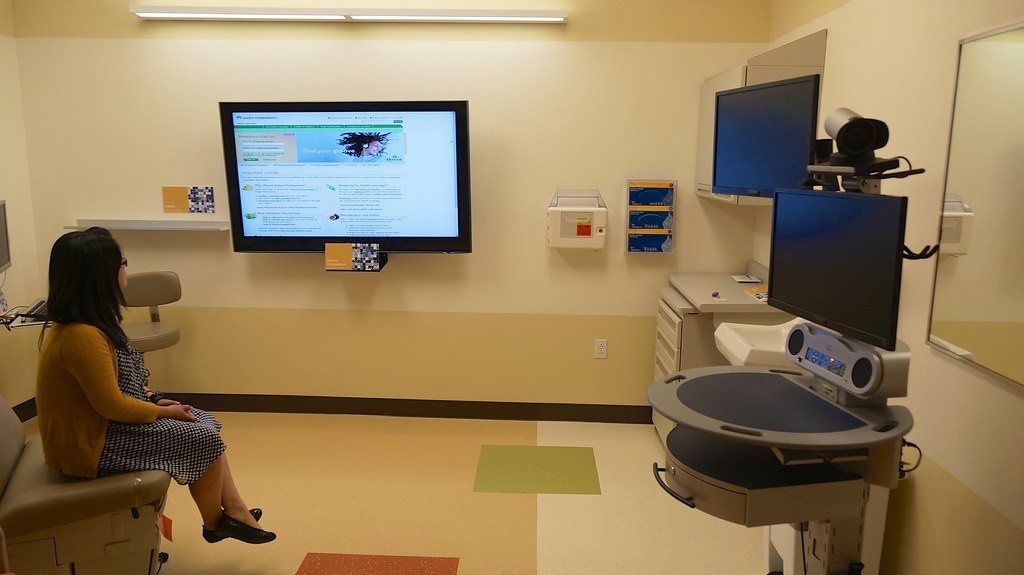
[20,297,49,319]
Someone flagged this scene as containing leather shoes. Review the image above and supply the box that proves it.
[202,508,262,543]
[217,515,276,544]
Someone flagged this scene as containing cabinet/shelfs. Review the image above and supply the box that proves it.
[652,258,797,447]
[695,63,747,205]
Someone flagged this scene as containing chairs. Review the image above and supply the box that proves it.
[0,394,169,575]
[121,270,181,354]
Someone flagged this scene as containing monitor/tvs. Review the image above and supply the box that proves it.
[219,101,472,254]
[766,187,908,353]
[0,200,12,276]
[711,74,820,198]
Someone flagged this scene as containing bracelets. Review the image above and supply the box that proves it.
[145,389,155,394]
[150,392,165,404]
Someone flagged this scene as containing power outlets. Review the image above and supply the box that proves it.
[594,338,607,359]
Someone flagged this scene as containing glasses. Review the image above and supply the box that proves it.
[120,257,127,267]
[372,142,381,148]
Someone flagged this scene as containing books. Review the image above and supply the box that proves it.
[733,275,768,302]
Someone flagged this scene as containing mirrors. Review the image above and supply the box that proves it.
[924,19,1024,393]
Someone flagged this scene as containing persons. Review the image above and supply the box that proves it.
[337,131,392,162]
[36,227,276,544]
